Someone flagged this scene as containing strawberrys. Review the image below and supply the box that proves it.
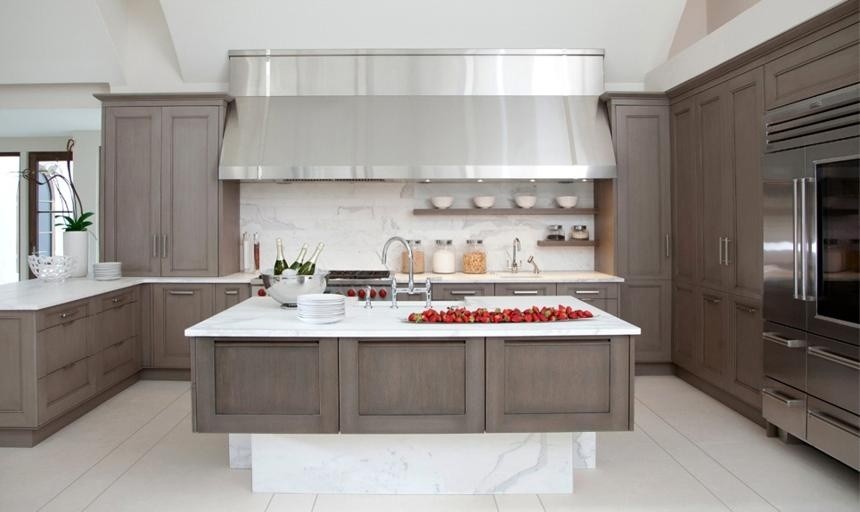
[409,304,594,323]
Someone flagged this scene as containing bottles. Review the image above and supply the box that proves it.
[823,236,859,273]
[289,244,308,275]
[463,240,488,273]
[297,243,325,275]
[571,225,589,240]
[275,238,288,275]
[402,241,423,273]
[241,230,261,271]
[433,239,458,274]
[545,225,566,240]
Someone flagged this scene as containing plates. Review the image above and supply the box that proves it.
[296,294,345,323]
[94,262,121,281]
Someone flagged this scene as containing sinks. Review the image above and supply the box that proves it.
[491,273,543,279]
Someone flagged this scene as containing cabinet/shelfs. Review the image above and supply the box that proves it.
[91,92,242,279]
[593,0,859,472]
[1,269,624,449]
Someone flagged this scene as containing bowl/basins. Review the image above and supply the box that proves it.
[555,196,579,208]
[514,195,537,208]
[472,197,496,209]
[260,273,329,307]
[433,197,453,209]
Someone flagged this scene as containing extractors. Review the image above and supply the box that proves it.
[216,52,615,187]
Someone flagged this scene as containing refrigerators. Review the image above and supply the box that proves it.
[763,83,858,467]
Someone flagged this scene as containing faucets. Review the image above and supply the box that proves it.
[512,237,522,268]
[380,235,414,292]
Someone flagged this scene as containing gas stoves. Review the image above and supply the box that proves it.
[326,269,393,300]
